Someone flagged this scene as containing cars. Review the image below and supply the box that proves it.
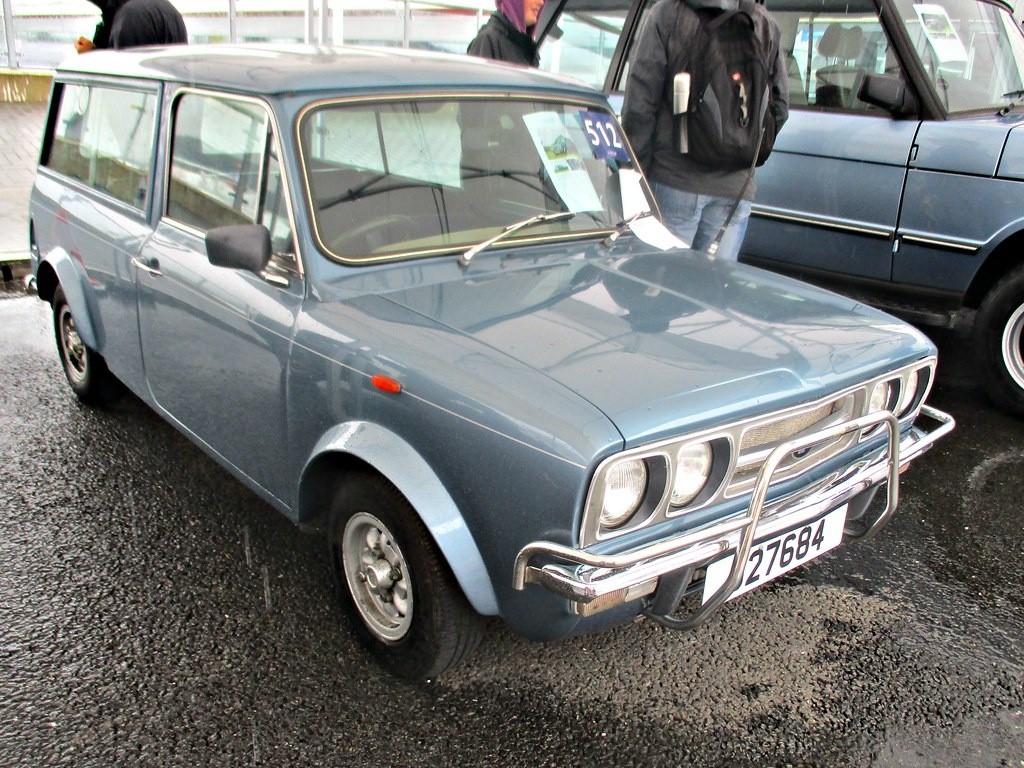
[527,1,1024,419]
[25,41,956,679]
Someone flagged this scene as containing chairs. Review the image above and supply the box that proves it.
[309,148,546,254]
[815,24,870,109]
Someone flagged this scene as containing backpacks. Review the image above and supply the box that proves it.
[670,0,777,175]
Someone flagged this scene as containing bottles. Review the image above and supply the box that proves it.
[673,73,690,115]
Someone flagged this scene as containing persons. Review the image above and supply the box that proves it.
[75,0,188,56]
[455,0,546,207]
[620,0,789,262]
